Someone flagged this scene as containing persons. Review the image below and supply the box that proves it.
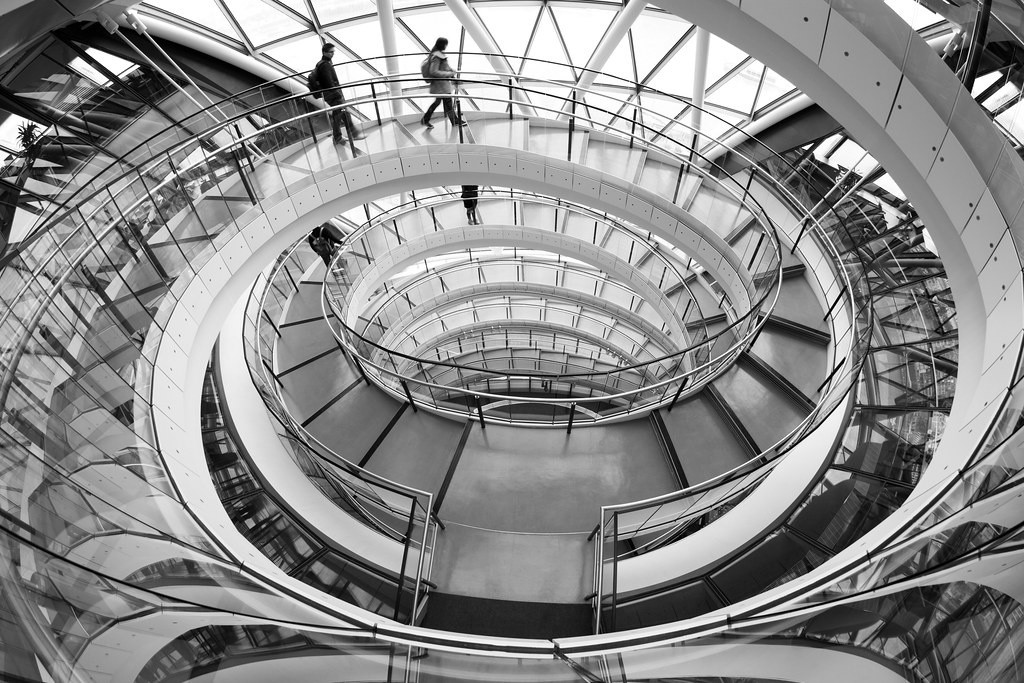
[309,226,347,276]
[420,37,466,128]
[462,185,481,225]
[315,43,368,144]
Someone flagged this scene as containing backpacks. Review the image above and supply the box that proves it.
[422,55,435,83]
[309,227,332,256]
[306,60,331,100]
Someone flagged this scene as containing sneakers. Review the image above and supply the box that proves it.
[452,119,467,125]
[421,119,434,128]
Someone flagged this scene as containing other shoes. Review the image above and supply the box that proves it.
[352,133,368,140]
[468,219,473,225]
[468,226,480,226]
[333,136,349,145]
[473,219,480,225]
